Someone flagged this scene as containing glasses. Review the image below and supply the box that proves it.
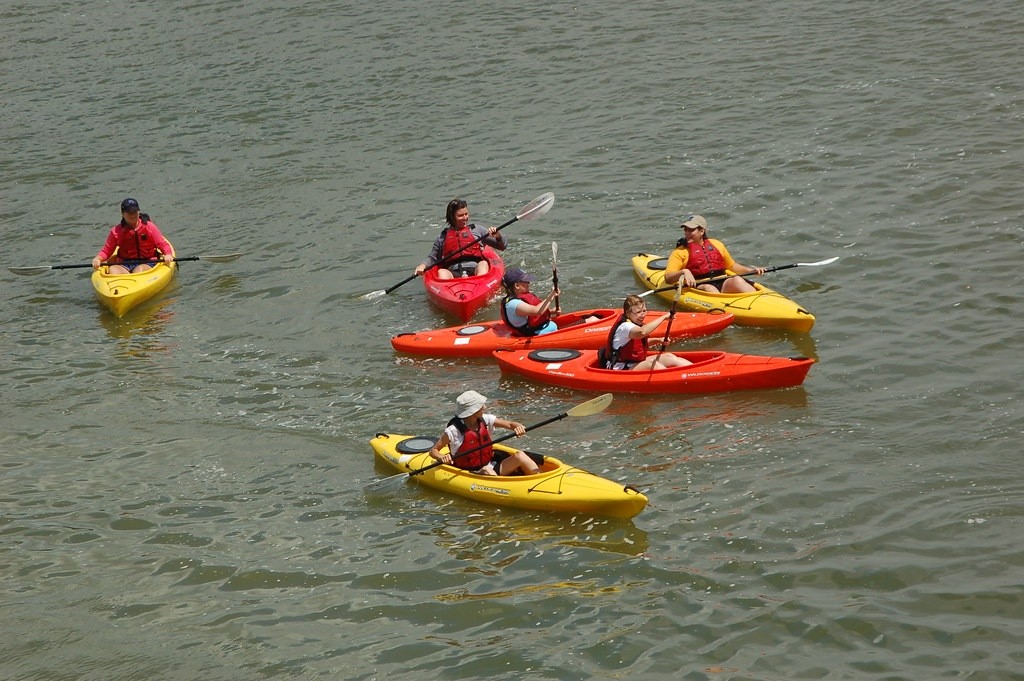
[450,201,466,206]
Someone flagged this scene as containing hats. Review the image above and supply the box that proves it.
[121,198,140,213]
[455,391,487,418]
[680,215,707,229]
[502,269,535,283]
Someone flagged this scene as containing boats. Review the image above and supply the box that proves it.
[368,432,648,522]
[630,251,816,334]
[390,307,735,357]
[90,236,177,317]
[422,246,506,321]
[494,348,816,393]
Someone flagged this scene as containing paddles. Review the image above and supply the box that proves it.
[634,256,842,296]
[551,240,560,313]
[359,189,554,297]
[660,300,679,352]
[362,392,613,496]
[9,254,242,278]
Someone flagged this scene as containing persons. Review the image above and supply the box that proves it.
[414,199,508,279]
[428,390,541,476]
[604,294,693,369]
[664,216,769,294]
[500,268,601,336]
[92,197,175,275]
